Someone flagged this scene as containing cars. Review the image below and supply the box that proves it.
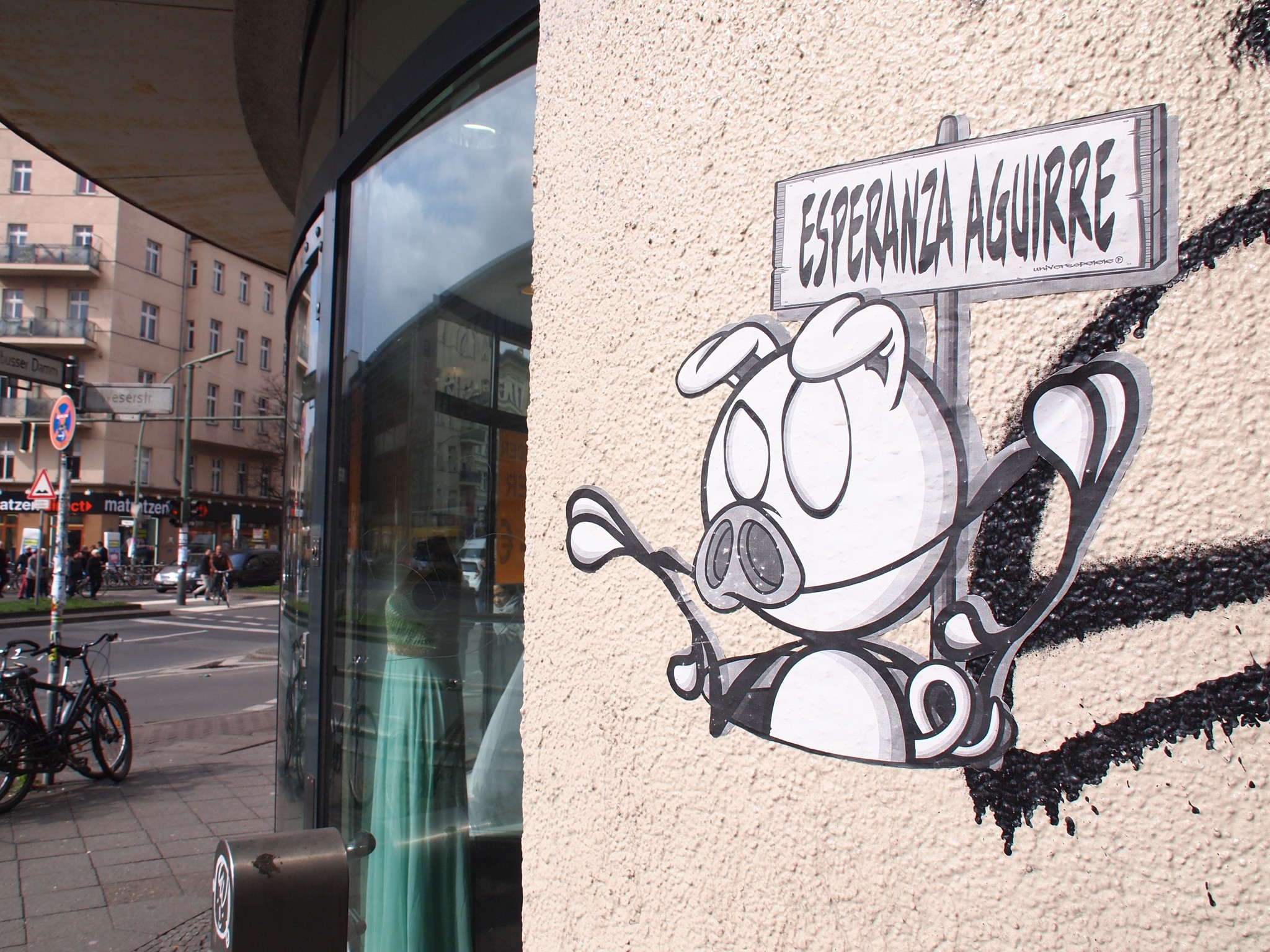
[455,558,487,596]
[153,553,213,594]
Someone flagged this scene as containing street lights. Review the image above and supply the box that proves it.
[130,347,236,581]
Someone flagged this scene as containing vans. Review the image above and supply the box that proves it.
[457,538,497,566]
[222,547,283,588]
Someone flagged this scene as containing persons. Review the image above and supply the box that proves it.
[0,537,111,603]
[361,530,477,952]
[473,547,525,743]
[204,545,233,602]
[193,549,218,602]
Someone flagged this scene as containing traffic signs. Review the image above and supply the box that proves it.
[83,382,174,415]
[0,342,66,386]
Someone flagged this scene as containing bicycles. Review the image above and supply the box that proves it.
[284,630,310,790]
[323,654,385,809]
[210,568,232,607]
[0,553,162,596]
[410,668,496,808]
[0,630,136,818]
